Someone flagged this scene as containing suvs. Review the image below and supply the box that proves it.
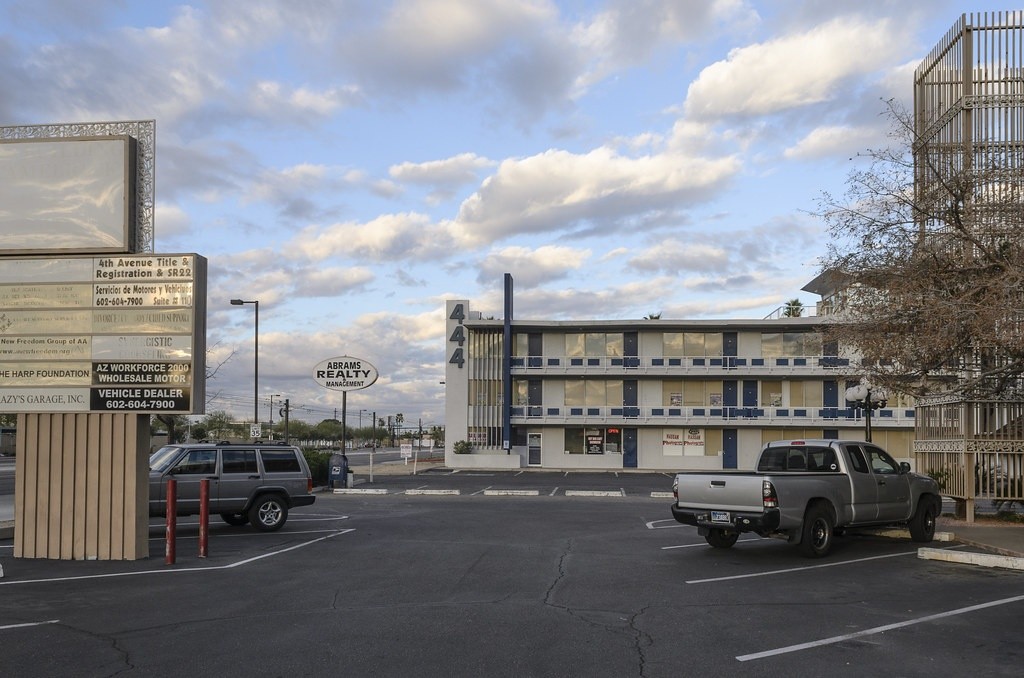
[149,441,317,532]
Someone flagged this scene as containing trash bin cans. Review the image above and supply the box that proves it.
[327,454,349,488]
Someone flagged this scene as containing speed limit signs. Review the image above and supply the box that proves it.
[249,423,262,438]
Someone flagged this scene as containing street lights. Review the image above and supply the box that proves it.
[360,410,367,430]
[270,394,280,435]
[229,298,259,444]
[844,376,888,443]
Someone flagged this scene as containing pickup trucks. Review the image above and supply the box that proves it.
[670,440,942,558]
